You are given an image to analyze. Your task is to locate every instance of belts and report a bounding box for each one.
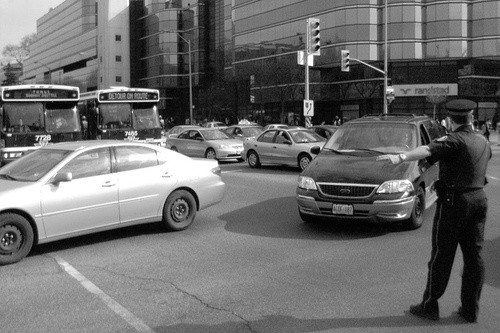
[455,188,478,193]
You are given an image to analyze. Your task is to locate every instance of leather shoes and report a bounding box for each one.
[409,304,439,321]
[458,306,477,323]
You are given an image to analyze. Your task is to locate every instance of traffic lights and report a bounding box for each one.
[308,17,320,56]
[340,49,350,72]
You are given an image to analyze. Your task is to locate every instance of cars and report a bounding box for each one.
[162,123,343,173]
[0,138,226,264]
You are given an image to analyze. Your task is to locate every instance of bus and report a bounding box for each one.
[77,84,164,148]
[0,83,89,167]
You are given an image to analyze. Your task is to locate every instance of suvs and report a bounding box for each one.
[294,111,453,232]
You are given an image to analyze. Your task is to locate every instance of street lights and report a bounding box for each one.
[80,52,99,90]
[33,61,51,84]
[166,31,193,126]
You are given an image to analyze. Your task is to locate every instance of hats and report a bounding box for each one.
[440,98,478,117]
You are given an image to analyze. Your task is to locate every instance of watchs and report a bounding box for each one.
[399,153,406,161]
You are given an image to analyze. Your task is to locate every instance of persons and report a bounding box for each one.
[255,112,304,127]
[422,113,492,145]
[185,115,230,126]
[158,112,175,132]
[376,100,492,322]
[334,115,341,125]
